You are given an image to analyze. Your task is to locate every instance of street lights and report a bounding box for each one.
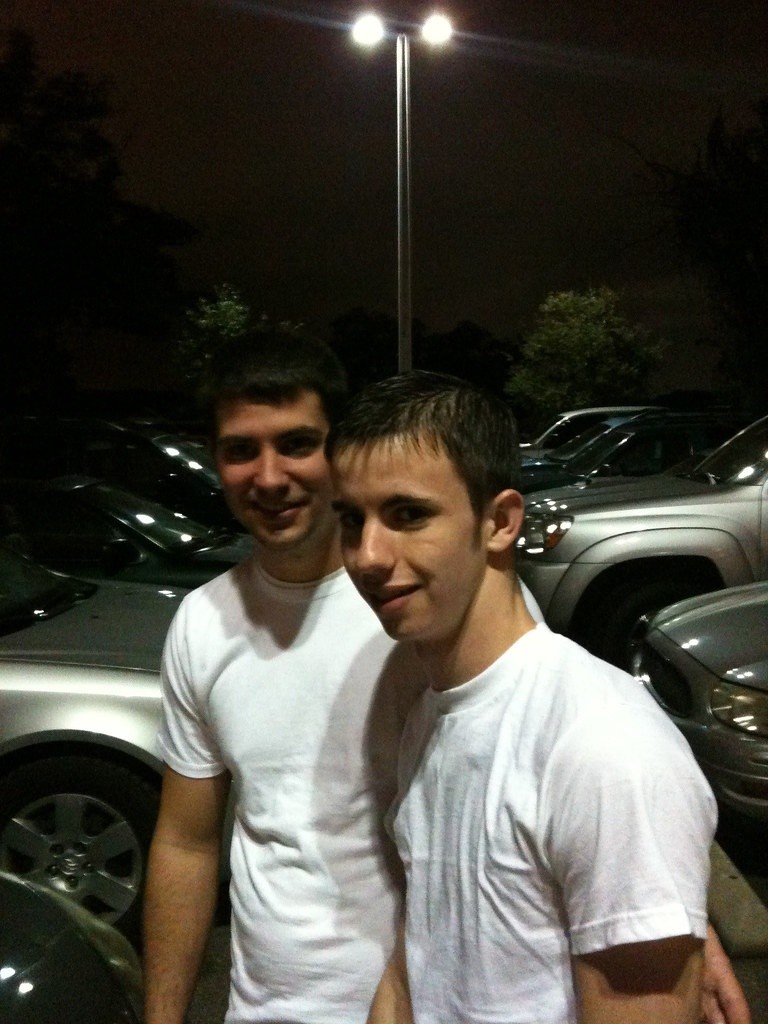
[352,3,460,373]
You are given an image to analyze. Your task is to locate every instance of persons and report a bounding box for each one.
[142,347,753,1024]
[325,370,720,1024]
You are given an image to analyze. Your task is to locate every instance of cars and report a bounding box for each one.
[630,577,768,836]
[514,403,768,675]
[1,402,267,1024]
